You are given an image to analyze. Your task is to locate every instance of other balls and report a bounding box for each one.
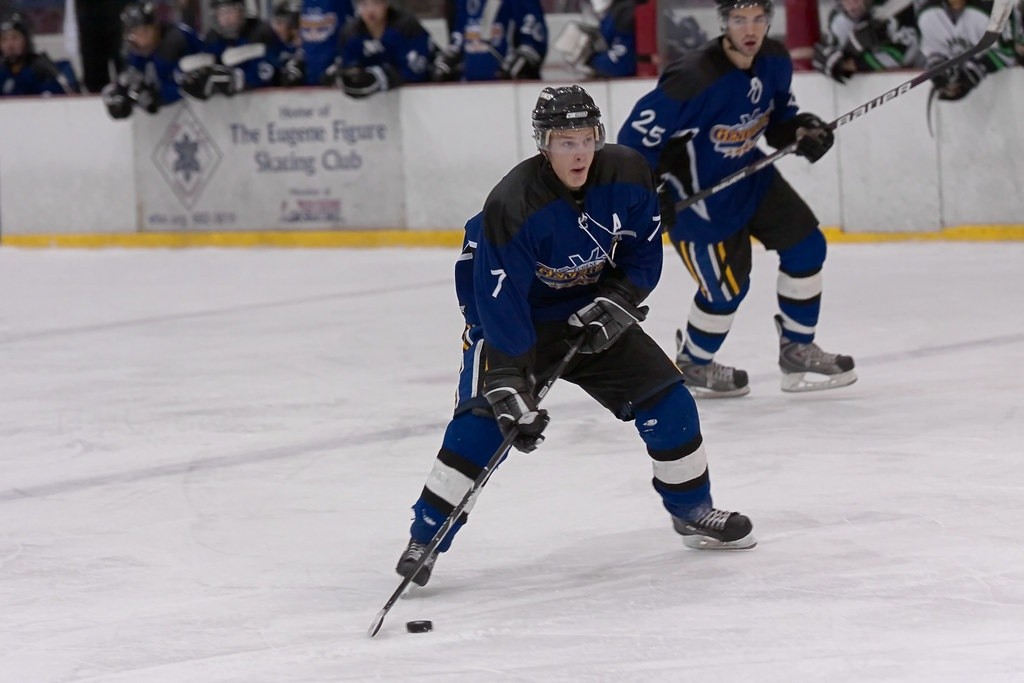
[406,620,433,633]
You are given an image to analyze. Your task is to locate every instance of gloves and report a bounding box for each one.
[432,56,462,81]
[566,293,649,355]
[184,65,232,100]
[928,53,983,102]
[502,47,542,79]
[481,368,549,453]
[104,72,159,120]
[767,104,835,163]
[652,172,675,227]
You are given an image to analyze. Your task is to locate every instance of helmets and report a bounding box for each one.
[715,0,774,35]
[208,0,244,37]
[120,0,160,58]
[532,84,608,161]
[0,9,33,65]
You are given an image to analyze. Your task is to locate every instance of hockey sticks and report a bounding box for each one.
[676,0,1014,213]
[368,330,588,638]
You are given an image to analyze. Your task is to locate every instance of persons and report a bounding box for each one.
[102,0,1024,122]
[396,85,754,586]
[0,18,81,99]
[617,0,856,392]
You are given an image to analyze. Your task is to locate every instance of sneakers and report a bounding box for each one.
[395,535,439,598]
[674,328,752,398]
[340,68,376,96]
[774,312,858,392]
[672,494,756,552]
